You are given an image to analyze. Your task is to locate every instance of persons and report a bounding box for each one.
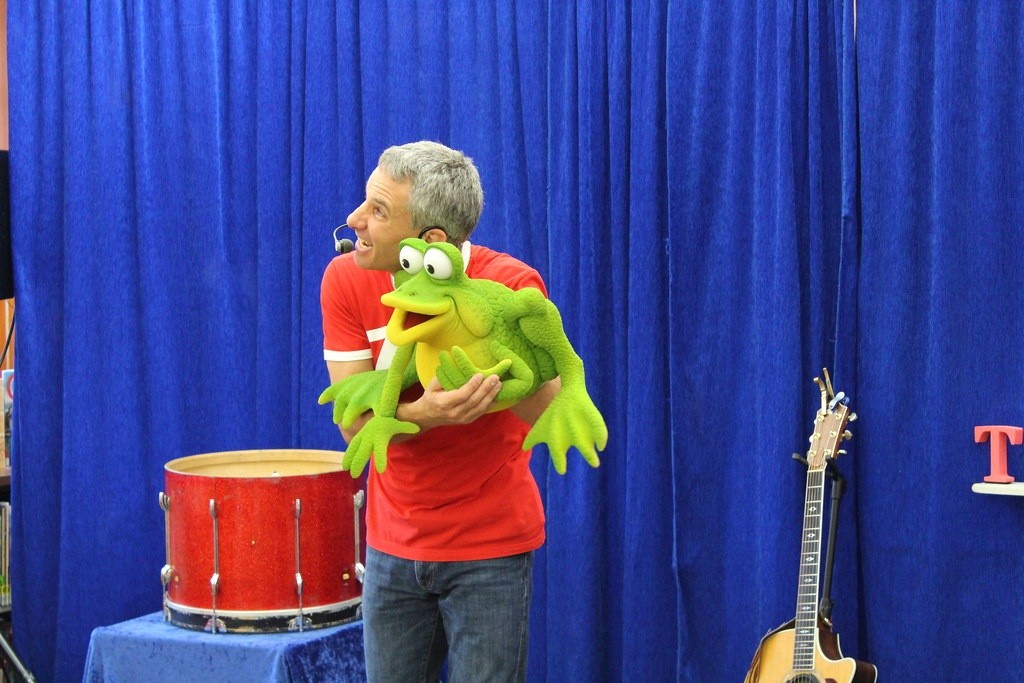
[320,140,562,683]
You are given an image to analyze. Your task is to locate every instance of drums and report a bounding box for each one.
[159,449,367,635]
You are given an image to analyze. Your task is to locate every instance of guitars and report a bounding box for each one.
[744,367,879,683]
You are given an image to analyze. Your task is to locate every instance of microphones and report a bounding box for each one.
[332,223,354,253]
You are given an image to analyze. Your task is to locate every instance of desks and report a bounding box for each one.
[82,607,368,682]
